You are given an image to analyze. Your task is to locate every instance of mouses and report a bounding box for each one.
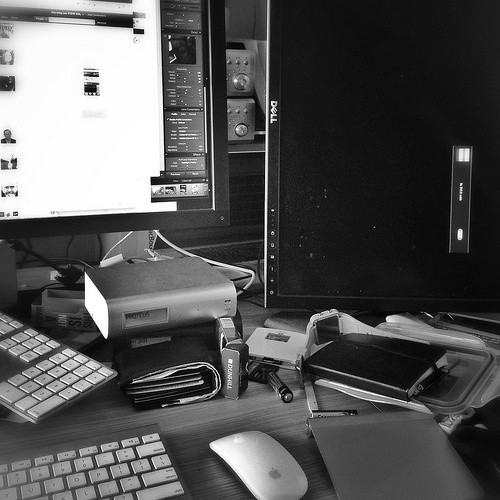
[207,431,309,500]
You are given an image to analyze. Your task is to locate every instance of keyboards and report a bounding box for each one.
[0,311,118,423]
[0,423,193,500]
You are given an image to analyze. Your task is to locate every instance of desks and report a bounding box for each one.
[0,297,499,500]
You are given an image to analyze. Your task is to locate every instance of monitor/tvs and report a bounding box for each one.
[264,0,500,312]
[0,0,231,264]
